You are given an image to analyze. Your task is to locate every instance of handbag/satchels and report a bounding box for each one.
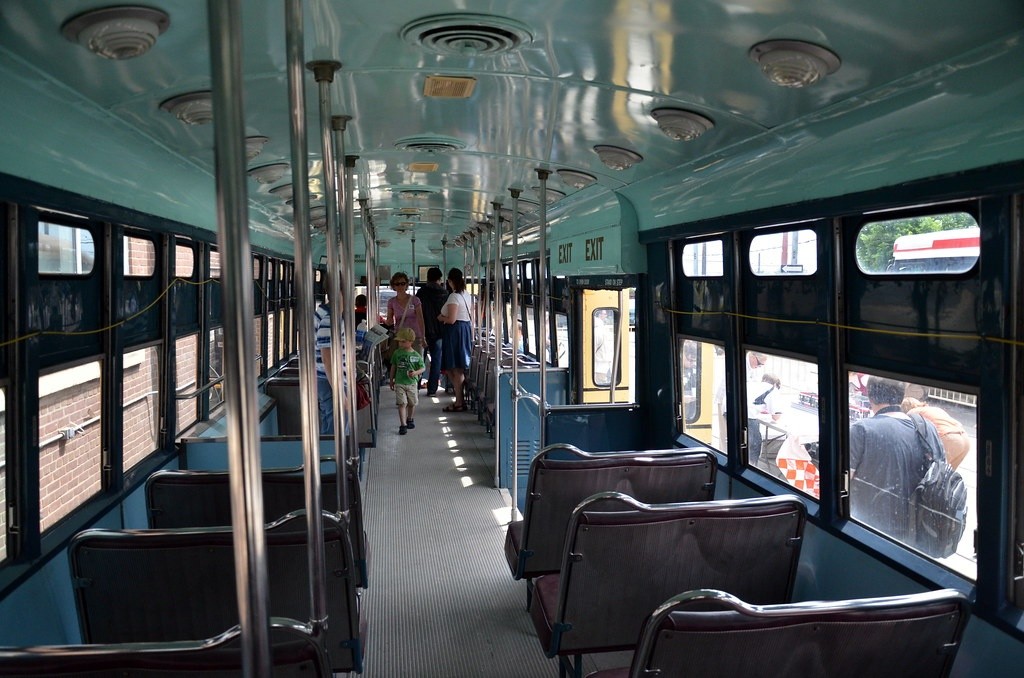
[343,382,370,412]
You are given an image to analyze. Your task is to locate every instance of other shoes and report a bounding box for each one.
[406,417,415,429]
[462,404,467,410]
[399,424,408,435]
[443,403,463,412]
[427,391,436,397]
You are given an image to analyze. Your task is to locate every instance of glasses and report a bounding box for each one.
[394,282,407,286]
[755,357,763,367]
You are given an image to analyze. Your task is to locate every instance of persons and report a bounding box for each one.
[849,375,946,543]
[716,350,768,453]
[437,267,471,412]
[747,372,781,466]
[901,397,971,471]
[415,268,449,396]
[382,272,428,404]
[389,327,426,434]
[313,276,350,435]
[354,295,383,323]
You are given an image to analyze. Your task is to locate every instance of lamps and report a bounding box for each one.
[532,187,566,204]
[247,163,290,184]
[158,90,213,126]
[59,4,171,60]
[650,107,715,143]
[748,39,841,86]
[556,169,597,188]
[246,136,269,161]
[593,145,643,171]
[268,184,292,196]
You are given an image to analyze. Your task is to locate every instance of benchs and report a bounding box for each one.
[0,356,380,678]
[468,338,970,678]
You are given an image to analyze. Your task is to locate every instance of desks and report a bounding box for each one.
[758,407,818,476]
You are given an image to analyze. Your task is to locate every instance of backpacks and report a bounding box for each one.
[907,412,969,560]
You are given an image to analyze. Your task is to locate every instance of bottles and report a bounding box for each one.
[356,319,367,346]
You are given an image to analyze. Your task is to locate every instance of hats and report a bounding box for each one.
[393,328,415,341]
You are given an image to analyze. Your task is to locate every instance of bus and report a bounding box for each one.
[490,287,714,446]
